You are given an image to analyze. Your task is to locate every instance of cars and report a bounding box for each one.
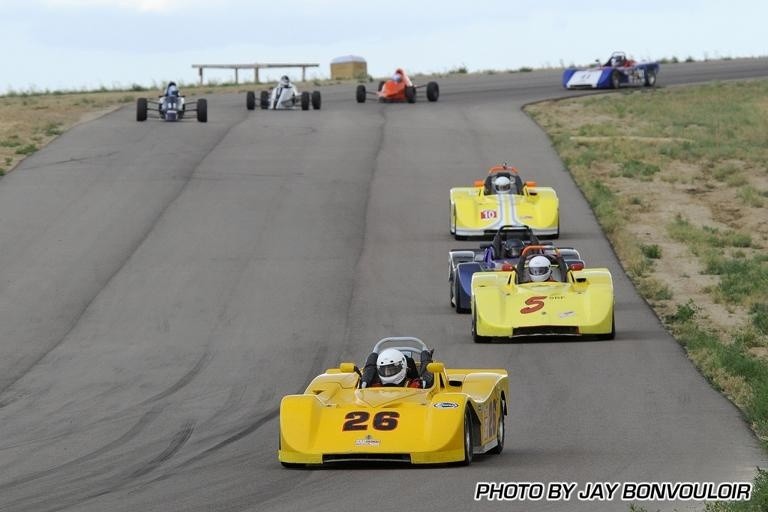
[277,336,510,470]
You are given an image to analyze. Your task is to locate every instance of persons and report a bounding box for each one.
[493,176,513,194]
[277,76,295,88]
[519,256,558,283]
[394,68,412,85]
[164,82,179,97]
[368,347,420,390]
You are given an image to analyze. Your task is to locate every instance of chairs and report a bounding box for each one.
[488,172,554,282]
[373,356,420,382]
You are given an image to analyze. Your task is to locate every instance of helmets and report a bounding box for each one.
[495,176,512,194]
[376,349,409,386]
[528,255,552,282]
[279,74,289,86]
[168,85,178,97]
